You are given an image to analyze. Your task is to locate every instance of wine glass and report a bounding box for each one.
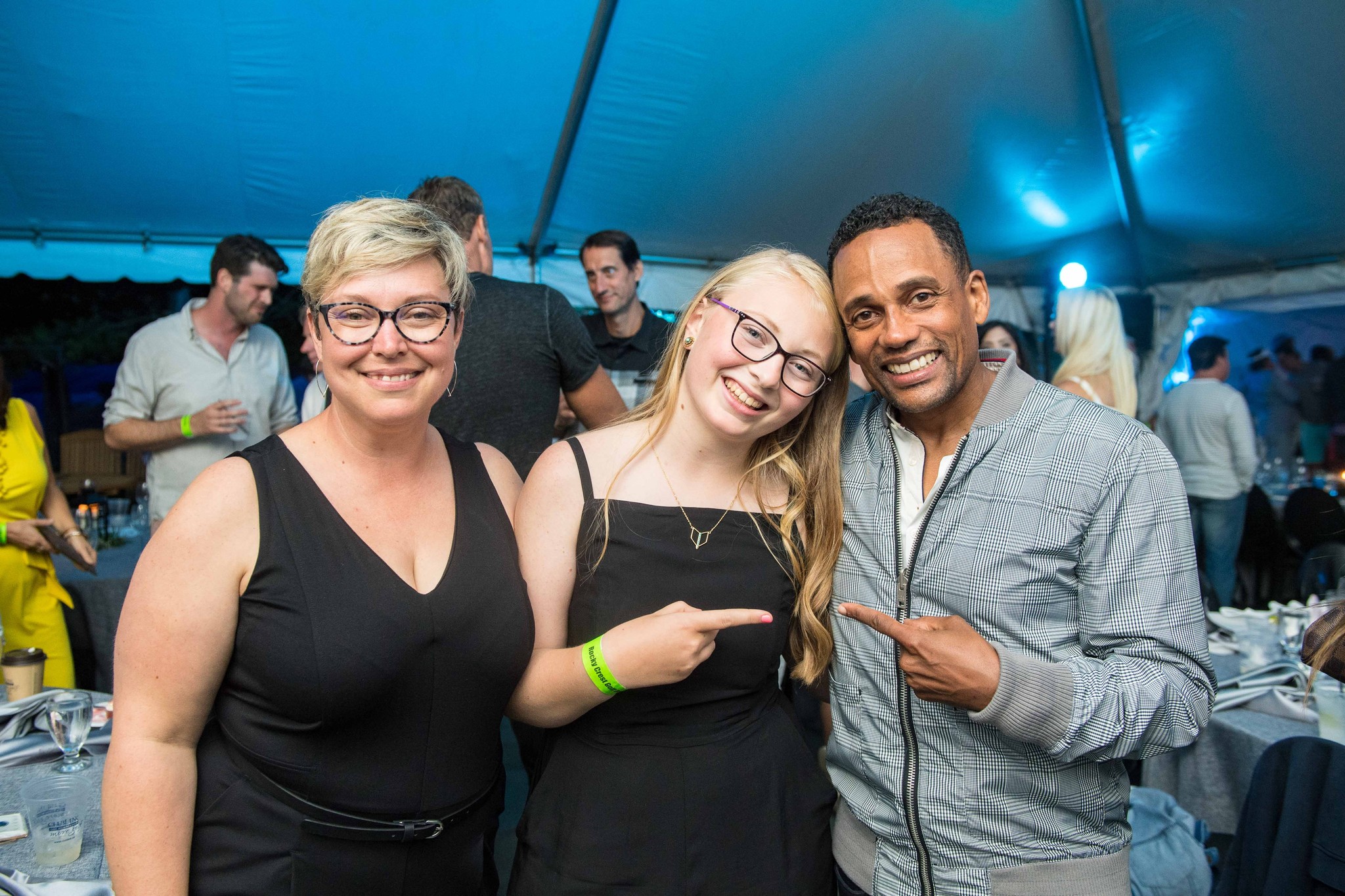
[1278,607,1310,659]
[45,691,94,774]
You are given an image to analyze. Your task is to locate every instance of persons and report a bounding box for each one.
[978,318,1029,372]
[101,228,301,545]
[1199,482,1345,613]
[552,229,678,437]
[1155,337,1261,608]
[290,305,333,424]
[406,177,632,480]
[0,375,96,692]
[1045,282,1140,416]
[1246,332,1344,479]
[826,195,1215,895]
[516,246,850,896]
[97,197,524,896]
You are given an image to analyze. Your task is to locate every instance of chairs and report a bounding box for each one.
[1238,481,1345,610]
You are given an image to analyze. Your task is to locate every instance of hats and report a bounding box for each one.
[1246,345,1272,363]
[1268,333,1295,354]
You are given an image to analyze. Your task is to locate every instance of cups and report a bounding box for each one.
[19,774,93,867]
[107,499,130,528]
[0,646,48,704]
[1311,679,1345,746]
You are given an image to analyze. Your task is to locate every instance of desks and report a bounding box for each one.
[39,509,150,695]
[1141,653,1345,834]
[0,684,115,896]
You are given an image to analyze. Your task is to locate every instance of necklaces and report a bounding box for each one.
[646,413,756,549]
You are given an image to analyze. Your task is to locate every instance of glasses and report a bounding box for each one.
[705,297,832,399]
[313,300,461,346]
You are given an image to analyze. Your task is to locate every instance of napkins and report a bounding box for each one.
[1206,594,1327,723]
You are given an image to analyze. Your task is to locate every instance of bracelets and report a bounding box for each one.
[581,632,629,698]
[177,414,197,440]
[59,525,86,540]
[0,523,8,545]
